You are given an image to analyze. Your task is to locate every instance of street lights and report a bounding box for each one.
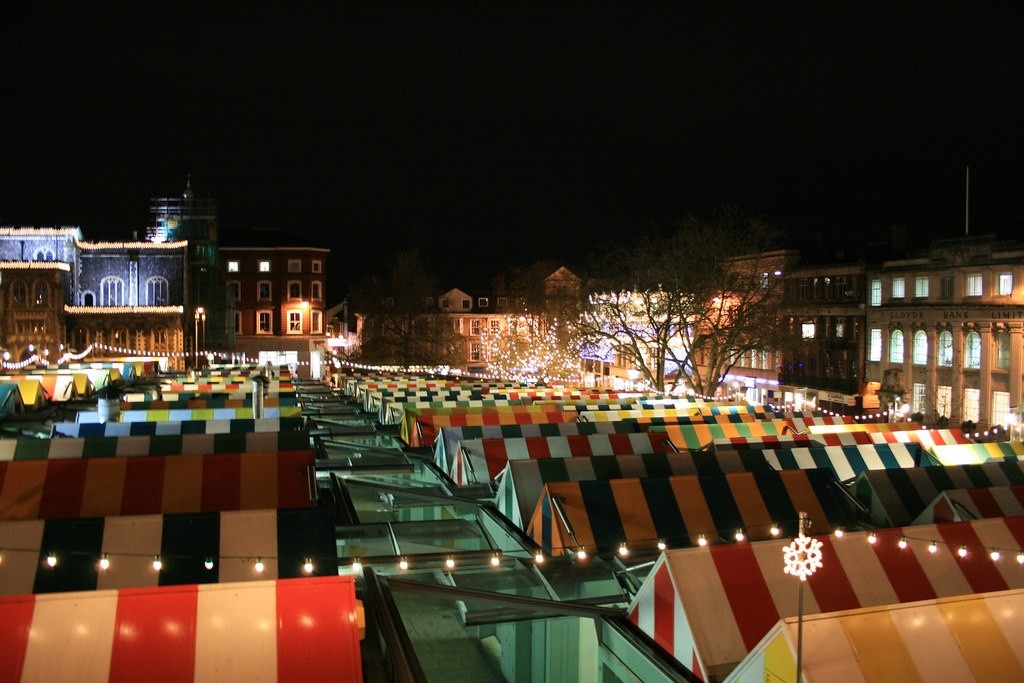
[195,307,206,372]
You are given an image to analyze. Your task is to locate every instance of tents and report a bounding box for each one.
[0,360,1024,683]
